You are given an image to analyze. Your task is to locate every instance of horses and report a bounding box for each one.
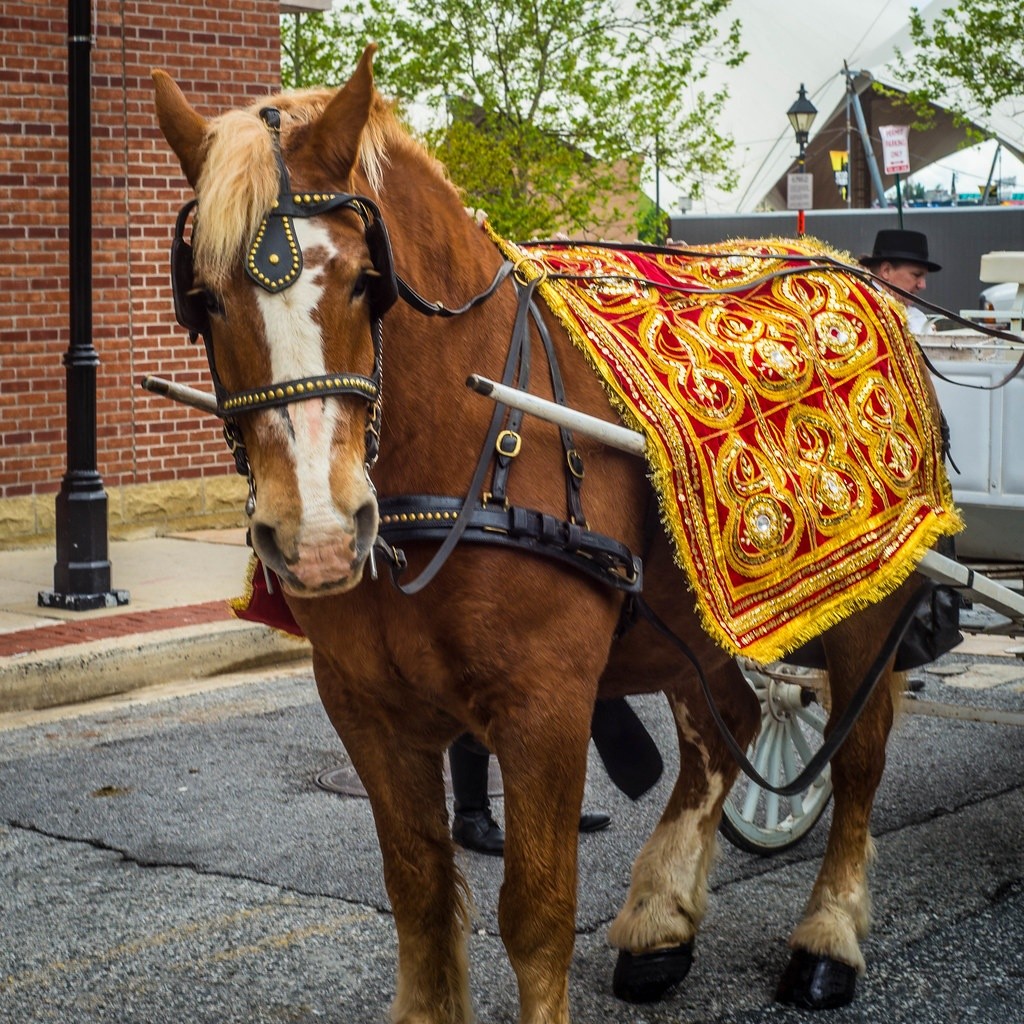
[151,43,942,1024]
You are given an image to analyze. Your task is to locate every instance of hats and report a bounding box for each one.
[858,229,943,273]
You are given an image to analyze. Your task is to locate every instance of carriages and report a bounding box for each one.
[144,39,1024,1024]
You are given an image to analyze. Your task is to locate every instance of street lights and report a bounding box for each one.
[786,81,819,173]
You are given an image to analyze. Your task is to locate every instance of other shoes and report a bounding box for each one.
[578,811,612,832]
[452,797,506,857]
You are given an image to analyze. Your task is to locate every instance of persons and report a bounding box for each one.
[447,732,612,858]
[855,230,942,336]
